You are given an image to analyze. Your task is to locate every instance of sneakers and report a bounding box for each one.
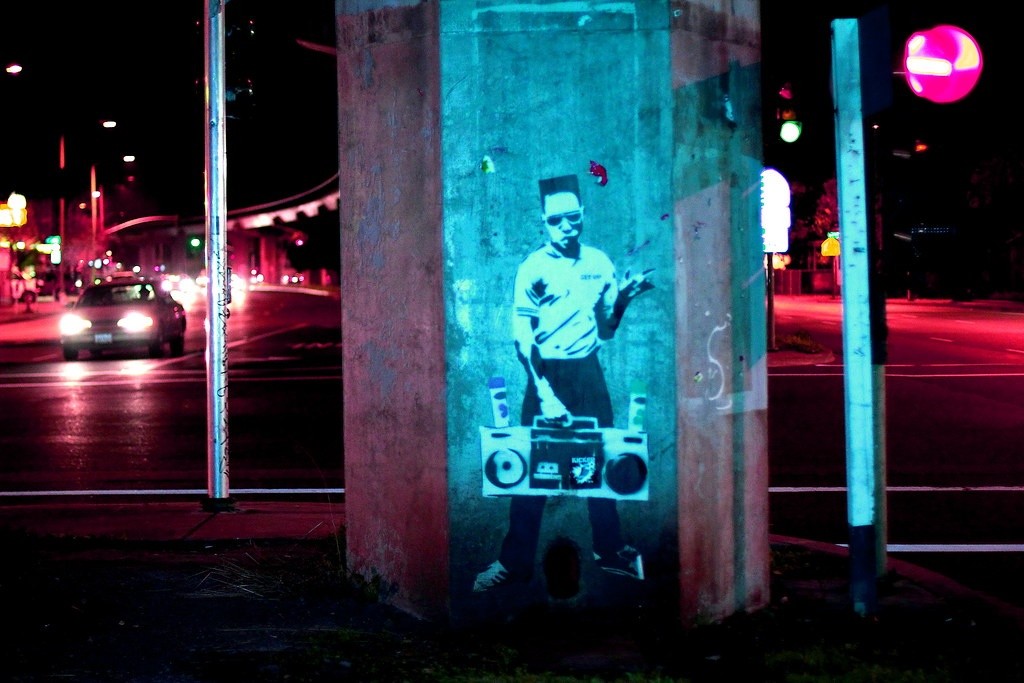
[593,545,644,580]
[475,560,510,590]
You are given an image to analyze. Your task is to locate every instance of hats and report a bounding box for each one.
[538,174,582,213]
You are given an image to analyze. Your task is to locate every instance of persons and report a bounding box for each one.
[471,174,656,595]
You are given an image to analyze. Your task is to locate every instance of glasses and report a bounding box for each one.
[544,208,582,226]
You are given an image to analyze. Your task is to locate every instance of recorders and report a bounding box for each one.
[478,425,649,500]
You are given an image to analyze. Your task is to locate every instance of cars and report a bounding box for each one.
[60,274,188,359]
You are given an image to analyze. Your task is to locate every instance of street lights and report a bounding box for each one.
[59,114,118,237]
